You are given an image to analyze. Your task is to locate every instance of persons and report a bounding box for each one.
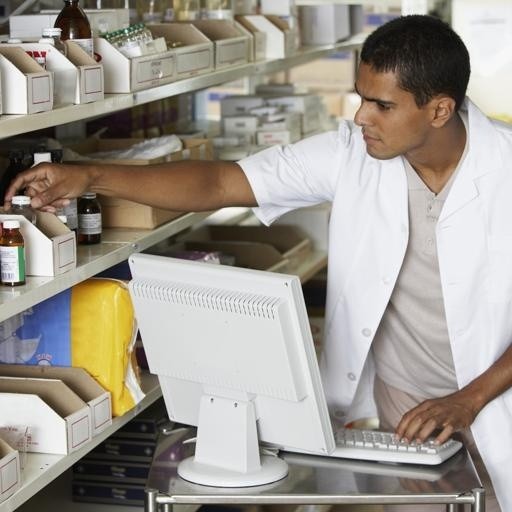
[4,14,512,512]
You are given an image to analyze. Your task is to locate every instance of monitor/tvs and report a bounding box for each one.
[127,251,336,488]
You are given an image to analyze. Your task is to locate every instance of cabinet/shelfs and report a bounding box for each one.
[1,0,362,512]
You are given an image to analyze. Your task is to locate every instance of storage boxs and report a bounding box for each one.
[60,134,213,228]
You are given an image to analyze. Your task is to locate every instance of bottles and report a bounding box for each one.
[163,0,176,23]
[79,194,102,246]
[140,0,163,24]
[8,196,36,227]
[49,149,78,237]
[173,1,199,21]
[207,0,233,26]
[3,148,30,195]
[54,0,93,58]
[103,22,153,52]
[0,220,26,287]
[39,28,67,57]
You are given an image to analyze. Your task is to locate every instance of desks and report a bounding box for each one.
[147,426,485,512]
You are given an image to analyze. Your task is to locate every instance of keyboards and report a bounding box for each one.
[331,426,463,466]
